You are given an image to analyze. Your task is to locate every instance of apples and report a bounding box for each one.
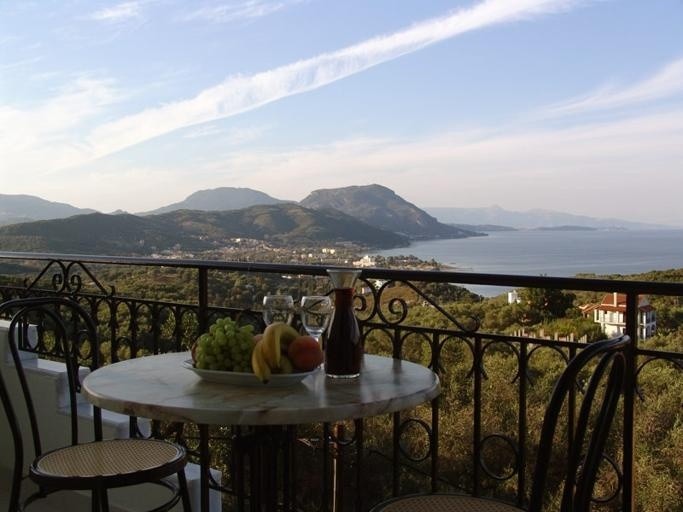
[289,336,321,371]
[191,336,199,362]
[254,335,263,344]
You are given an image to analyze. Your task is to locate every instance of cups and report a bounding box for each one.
[262,295,294,332]
[325,269,364,379]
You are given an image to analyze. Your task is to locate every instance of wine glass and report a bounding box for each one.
[300,296,331,371]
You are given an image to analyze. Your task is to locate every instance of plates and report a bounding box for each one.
[182,356,318,390]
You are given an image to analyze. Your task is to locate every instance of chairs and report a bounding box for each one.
[0,294,185,512]
[366,332,633,508]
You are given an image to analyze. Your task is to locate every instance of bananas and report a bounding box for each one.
[252,322,300,385]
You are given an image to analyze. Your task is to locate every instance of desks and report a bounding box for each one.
[81,345,442,512]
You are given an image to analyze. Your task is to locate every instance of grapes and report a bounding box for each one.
[194,317,255,373]
